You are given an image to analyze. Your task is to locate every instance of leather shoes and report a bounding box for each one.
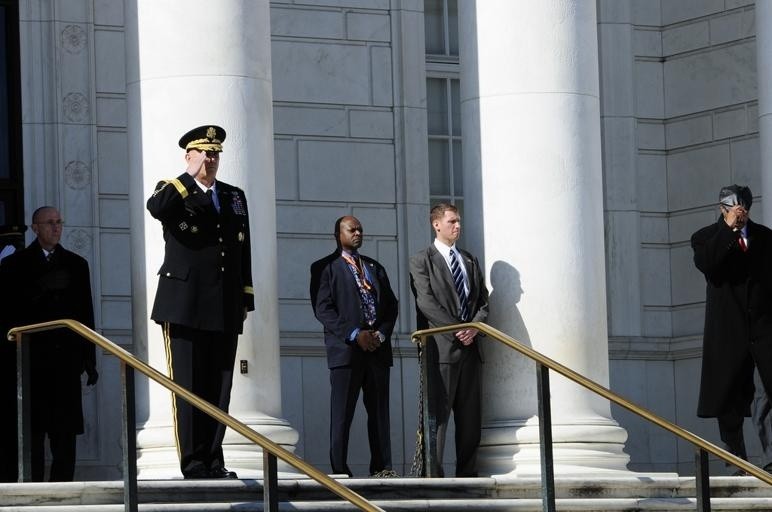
[86,366,99,387]
[0,244,16,261]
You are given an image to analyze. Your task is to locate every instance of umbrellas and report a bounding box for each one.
[209,467,237,480]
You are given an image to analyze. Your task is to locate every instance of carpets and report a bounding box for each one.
[205,189,216,210]
[351,259,377,324]
[448,249,469,320]
[45,252,55,263]
[735,230,748,252]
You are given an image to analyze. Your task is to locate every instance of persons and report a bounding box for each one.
[1,220,27,262]
[685,182,772,478]
[306,210,402,479]
[0,203,100,485]
[406,199,492,478]
[145,119,259,480]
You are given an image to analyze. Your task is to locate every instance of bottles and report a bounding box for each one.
[178,124,227,154]
[1,224,29,240]
[719,183,753,215]
